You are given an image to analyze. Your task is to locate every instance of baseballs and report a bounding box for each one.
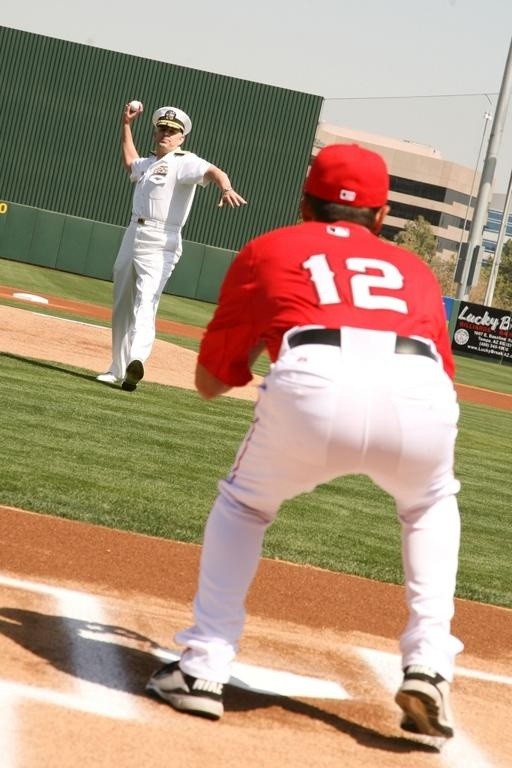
[129,100,140,112]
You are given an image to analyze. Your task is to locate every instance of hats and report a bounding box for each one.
[152,106,192,135]
[302,144,390,208]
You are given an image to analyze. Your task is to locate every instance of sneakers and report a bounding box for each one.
[145,659,224,721]
[96,360,144,392]
[395,663,454,739]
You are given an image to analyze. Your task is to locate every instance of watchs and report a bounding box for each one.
[220,184,232,194]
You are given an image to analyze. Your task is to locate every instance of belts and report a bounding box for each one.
[287,329,436,362]
[131,215,181,233]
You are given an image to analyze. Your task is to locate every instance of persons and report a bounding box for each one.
[93,102,250,392]
[145,142,466,739]
